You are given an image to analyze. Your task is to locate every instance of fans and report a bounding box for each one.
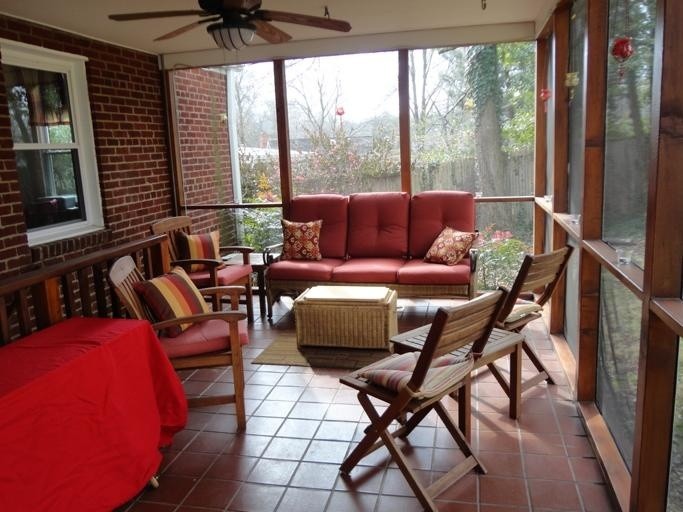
[107,0,352,46]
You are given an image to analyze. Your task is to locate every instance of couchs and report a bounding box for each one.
[263,192,478,322]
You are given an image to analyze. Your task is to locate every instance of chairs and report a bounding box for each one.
[337,284,508,511]
[449,244,576,421]
[150,215,256,321]
[106,254,247,435]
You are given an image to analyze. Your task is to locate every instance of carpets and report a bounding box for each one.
[251,330,391,369]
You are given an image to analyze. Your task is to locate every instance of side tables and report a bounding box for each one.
[391,319,525,452]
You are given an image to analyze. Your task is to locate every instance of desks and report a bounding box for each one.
[0,316,187,511]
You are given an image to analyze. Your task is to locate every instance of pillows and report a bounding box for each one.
[131,265,212,339]
[422,226,478,265]
[280,219,322,261]
[177,227,220,273]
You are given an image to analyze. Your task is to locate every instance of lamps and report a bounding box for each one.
[207,11,256,50]
